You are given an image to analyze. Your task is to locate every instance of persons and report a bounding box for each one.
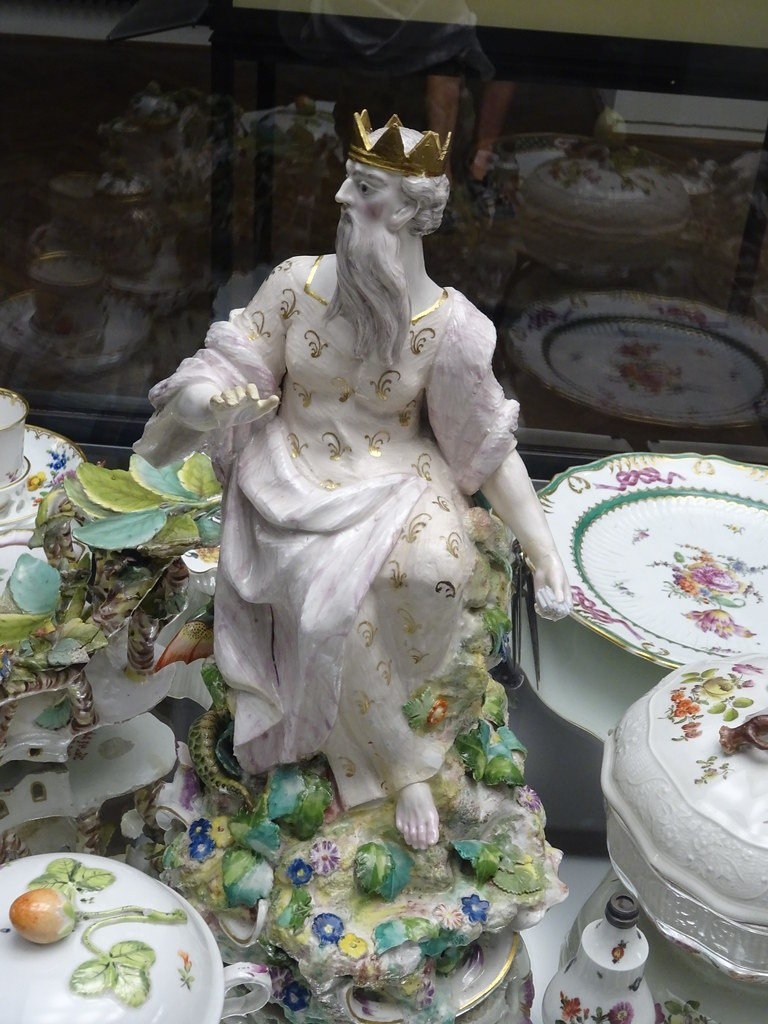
[151,116,576,851]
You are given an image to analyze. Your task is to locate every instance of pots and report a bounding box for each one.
[511,147,692,278]
[600,652,768,988]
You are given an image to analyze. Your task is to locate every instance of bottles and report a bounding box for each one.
[541,894,656,1024]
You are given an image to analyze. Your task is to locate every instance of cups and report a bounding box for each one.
[0,387,30,488]
[0,852,273,1024]
[27,252,110,335]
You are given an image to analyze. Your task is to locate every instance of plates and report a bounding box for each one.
[28,309,108,356]
[0,289,152,375]
[0,425,87,526]
[504,291,768,428]
[517,452,768,671]
[0,454,32,513]
